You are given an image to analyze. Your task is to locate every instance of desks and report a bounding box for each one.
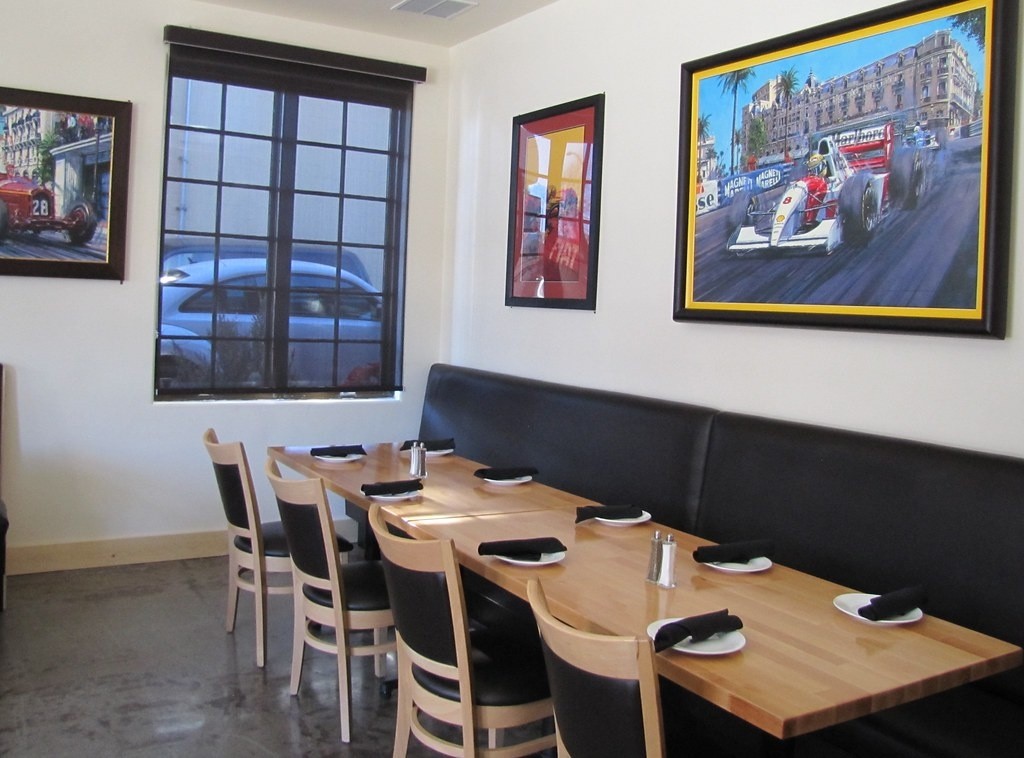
[267,446,605,530]
[405,504,1024,741]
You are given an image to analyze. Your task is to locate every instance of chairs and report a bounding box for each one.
[364,504,557,757]
[528,579,667,758]
[202,429,351,668]
[264,458,396,743]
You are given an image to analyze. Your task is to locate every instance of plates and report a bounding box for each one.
[482,475,532,487]
[833,593,923,627]
[703,556,773,575]
[647,617,746,655]
[493,552,566,567]
[593,509,651,528]
[370,490,421,502]
[314,454,363,464]
[426,449,454,458]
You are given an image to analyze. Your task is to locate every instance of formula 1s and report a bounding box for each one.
[723,120,929,258]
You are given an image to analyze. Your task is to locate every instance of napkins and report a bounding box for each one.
[311,444,368,457]
[362,479,423,496]
[475,466,539,481]
[476,536,566,562]
[400,437,454,452]
[692,538,776,563]
[575,504,642,522]
[858,582,931,623]
[653,608,744,654]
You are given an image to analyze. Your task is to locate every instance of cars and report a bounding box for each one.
[1,166,99,248]
[161,257,382,384]
[160,325,224,391]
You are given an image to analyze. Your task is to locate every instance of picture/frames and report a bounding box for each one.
[504,92,608,309]
[0,84,133,281]
[674,0,1018,340]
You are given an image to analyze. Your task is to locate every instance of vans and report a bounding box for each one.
[161,238,375,284]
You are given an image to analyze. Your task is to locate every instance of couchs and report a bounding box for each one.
[418,364,1024,756]
